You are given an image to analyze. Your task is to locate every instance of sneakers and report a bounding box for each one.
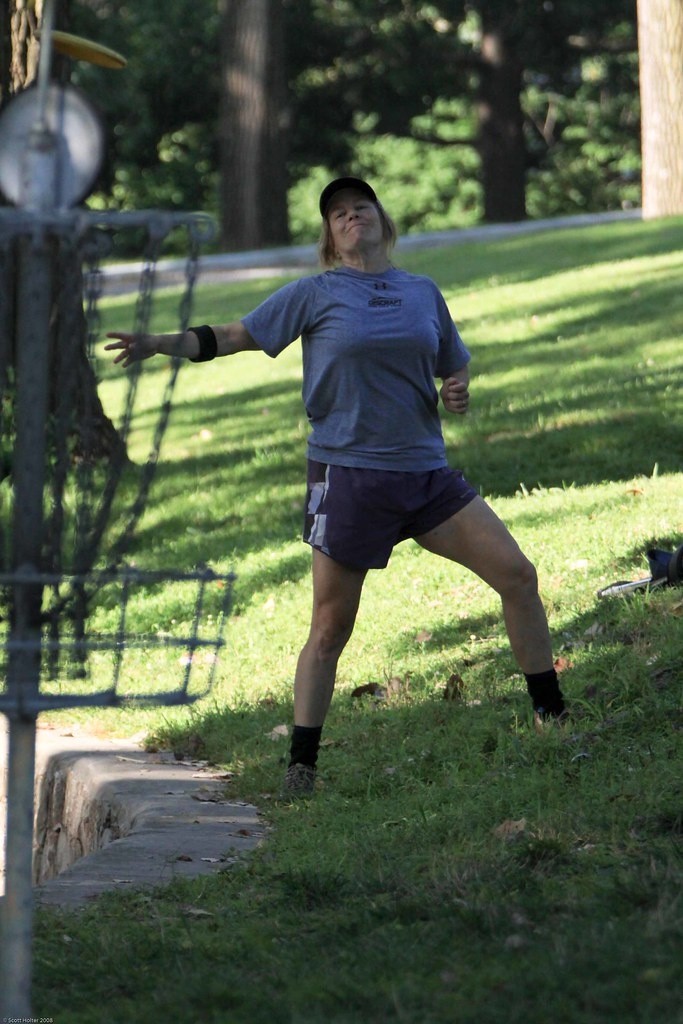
[280,765,317,805]
[537,705,586,732]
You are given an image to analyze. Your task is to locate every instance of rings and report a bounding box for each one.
[461,399,466,407]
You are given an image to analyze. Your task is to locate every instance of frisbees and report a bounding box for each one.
[29,28,125,69]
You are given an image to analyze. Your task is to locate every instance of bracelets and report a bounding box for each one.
[186,324,220,362]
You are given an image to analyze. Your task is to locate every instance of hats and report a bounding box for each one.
[320,178,375,217]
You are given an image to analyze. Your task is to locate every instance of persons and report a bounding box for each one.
[102,176,578,805]
